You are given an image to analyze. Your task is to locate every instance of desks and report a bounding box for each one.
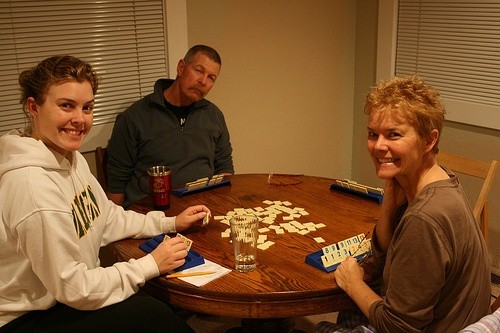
[109,173,393,333]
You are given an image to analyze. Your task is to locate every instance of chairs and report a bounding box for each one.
[436,151,500,248]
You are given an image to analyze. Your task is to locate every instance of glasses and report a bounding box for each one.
[351,231,372,266]
[268,173,304,186]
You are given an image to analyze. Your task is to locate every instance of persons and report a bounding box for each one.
[315,75,491,333]
[106,44,234,206]
[0,55,211,333]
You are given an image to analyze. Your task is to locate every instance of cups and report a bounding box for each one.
[229,215,259,273]
[146,166,172,211]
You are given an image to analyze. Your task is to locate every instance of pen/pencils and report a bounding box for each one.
[166,271,216,278]
